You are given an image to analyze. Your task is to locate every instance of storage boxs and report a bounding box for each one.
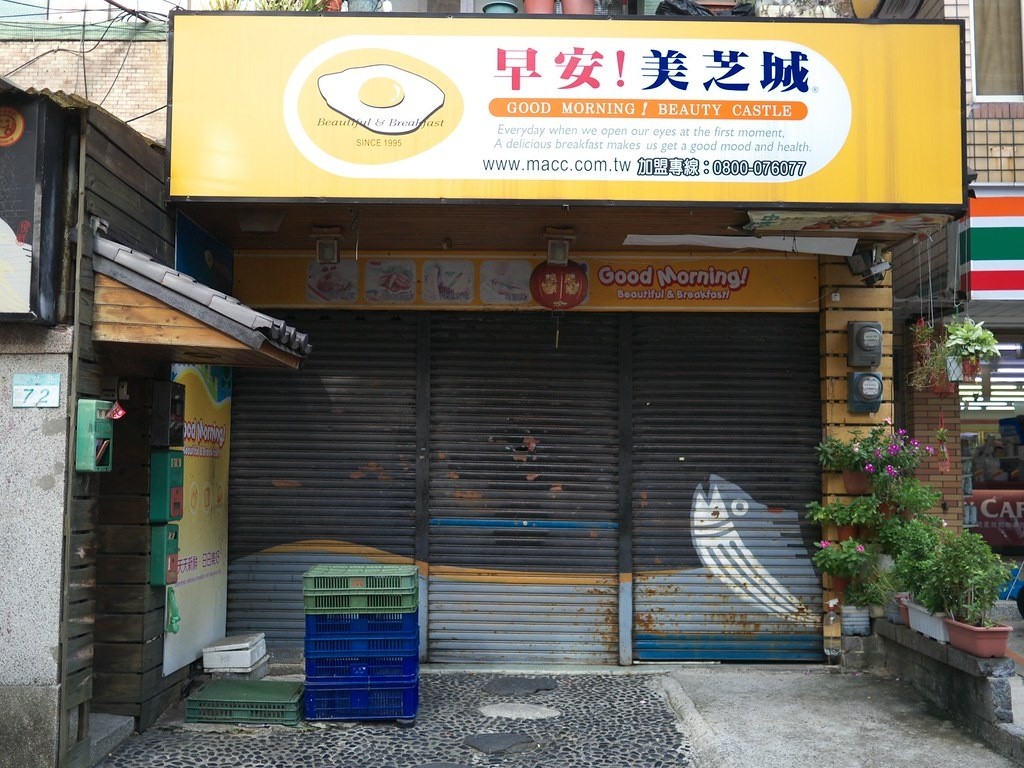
[185,563,420,727]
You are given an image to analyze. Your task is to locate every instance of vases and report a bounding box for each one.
[482,0,596,14]
[831,577,850,594]
[323,0,381,12]
[842,469,871,496]
[912,343,931,363]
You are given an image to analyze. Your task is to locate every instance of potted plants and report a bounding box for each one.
[905,312,1003,393]
[804,476,1024,658]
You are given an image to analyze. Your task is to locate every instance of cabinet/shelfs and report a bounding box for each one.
[961,457,980,527]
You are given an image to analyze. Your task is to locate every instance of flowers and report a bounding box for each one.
[910,318,935,343]
[812,535,872,578]
[863,427,936,497]
[816,416,893,473]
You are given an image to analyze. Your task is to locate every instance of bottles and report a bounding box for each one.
[823,608,841,656]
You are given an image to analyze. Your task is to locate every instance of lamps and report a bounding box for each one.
[543,227,577,267]
[309,226,347,264]
[847,242,894,287]
